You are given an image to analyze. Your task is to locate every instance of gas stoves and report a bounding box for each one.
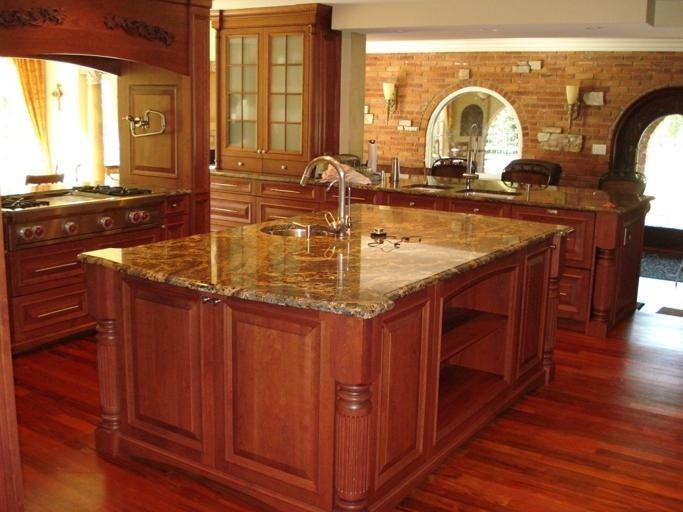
[0,184,169,253]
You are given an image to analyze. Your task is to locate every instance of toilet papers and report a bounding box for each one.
[367,142,377,174]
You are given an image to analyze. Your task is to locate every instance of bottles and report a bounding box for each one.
[360,138,401,187]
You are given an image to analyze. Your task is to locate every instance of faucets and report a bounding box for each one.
[300,155,347,235]
[462,123,479,190]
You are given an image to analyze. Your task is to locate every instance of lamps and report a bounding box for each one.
[565,86,579,133]
[382,83,397,125]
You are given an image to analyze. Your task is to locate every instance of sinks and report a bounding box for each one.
[399,183,456,192]
[455,189,522,198]
[259,224,345,237]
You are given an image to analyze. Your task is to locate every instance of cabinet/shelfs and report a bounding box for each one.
[209,3,341,178]
[7,196,198,357]
[212,179,596,330]
[370,241,560,499]
[122,277,330,509]
